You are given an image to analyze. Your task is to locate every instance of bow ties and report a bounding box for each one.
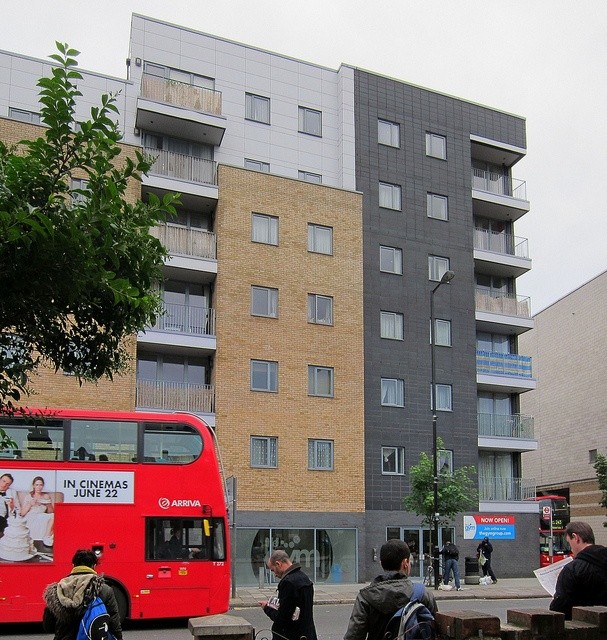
[0,492,6,497]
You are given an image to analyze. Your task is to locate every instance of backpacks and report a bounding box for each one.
[77,597,118,640]
[444,543,459,556]
[478,550,488,568]
[383,583,441,640]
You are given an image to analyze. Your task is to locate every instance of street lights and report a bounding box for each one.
[431,270,456,590]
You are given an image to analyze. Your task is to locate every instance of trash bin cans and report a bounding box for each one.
[464,556,480,585]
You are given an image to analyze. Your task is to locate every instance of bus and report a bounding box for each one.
[0,406,231,623]
[526,495,570,568]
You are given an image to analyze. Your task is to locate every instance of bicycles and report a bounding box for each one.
[422,552,453,587]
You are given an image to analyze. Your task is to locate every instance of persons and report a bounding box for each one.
[42,549,123,640]
[437,540,461,591]
[344,538,441,639]
[549,522,606,620]
[17,476,55,547]
[167,527,201,559]
[258,549,317,639]
[0,473,20,539]
[477,536,497,585]
